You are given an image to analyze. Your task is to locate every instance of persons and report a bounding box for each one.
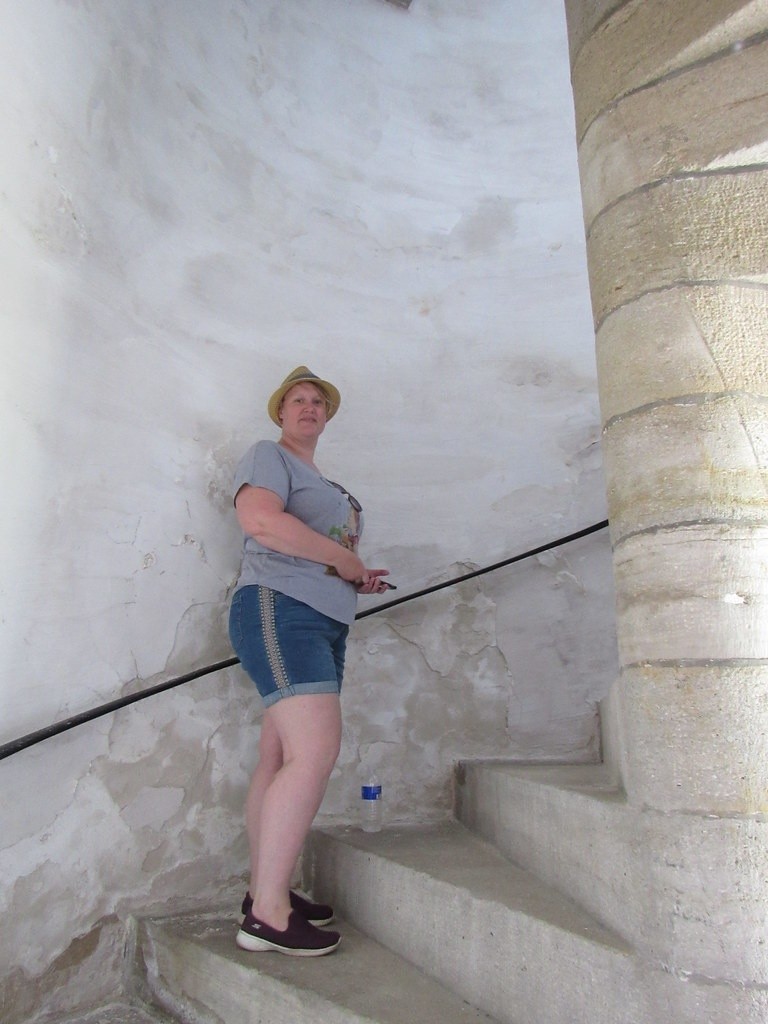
[228,365,390,957]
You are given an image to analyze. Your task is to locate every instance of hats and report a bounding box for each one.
[267,366,341,429]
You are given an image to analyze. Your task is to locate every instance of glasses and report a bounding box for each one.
[327,479,362,513]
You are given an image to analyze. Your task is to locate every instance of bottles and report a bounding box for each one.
[361,769,382,832]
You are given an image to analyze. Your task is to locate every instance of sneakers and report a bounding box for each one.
[236,907,342,958]
[238,888,333,927]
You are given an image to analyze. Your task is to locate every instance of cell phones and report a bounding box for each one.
[368,577,397,590]
